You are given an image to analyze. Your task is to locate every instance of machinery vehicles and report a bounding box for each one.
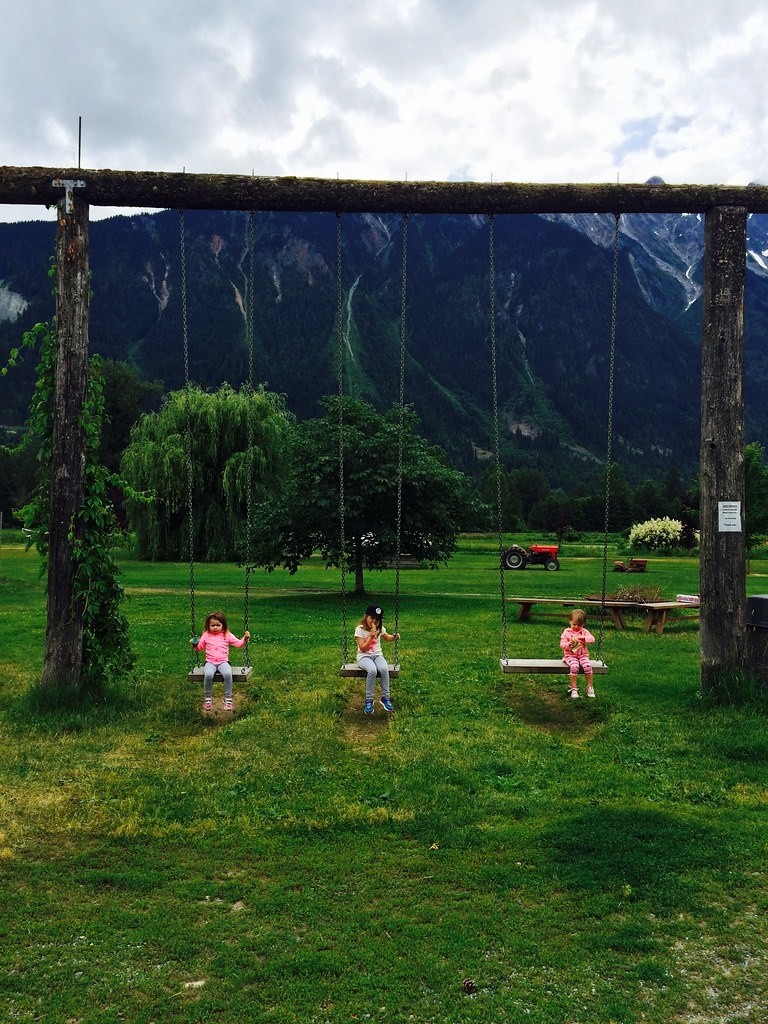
[500,544,560,571]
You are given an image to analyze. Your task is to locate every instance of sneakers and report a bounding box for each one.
[379,695,394,712]
[364,699,374,715]
[203,696,212,711]
[221,696,232,711]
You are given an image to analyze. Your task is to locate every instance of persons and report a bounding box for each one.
[189,611,250,712]
[354,606,401,715]
[560,609,596,698]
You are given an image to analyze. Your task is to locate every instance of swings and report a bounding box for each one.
[489,208,622,670]
[168,208,256,678]
[337,210,407,674]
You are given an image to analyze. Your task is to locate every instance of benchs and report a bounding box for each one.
[507,597,638,631]
[640,602,699,635]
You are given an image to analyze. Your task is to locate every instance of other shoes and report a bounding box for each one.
[585,685,596,698]
[567,687,580,699]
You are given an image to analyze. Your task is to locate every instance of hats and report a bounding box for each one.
[365,605,385,621]
